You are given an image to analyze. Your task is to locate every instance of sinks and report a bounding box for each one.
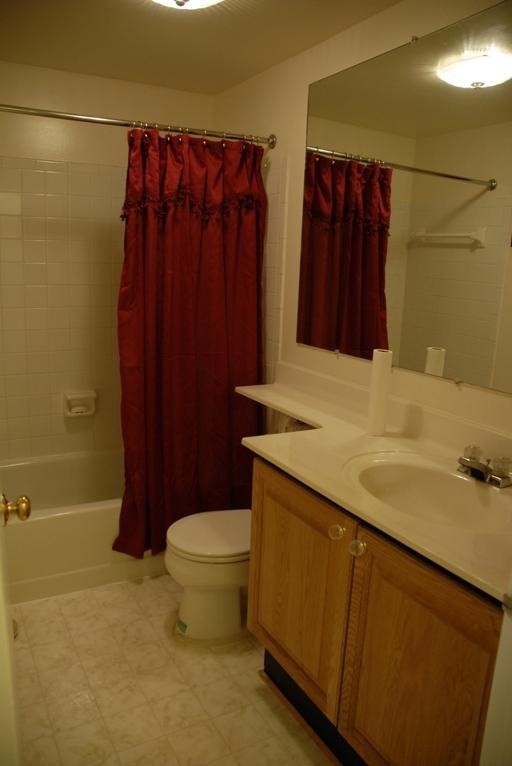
[342,451,512,532]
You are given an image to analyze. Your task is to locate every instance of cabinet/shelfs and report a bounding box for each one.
[246,460,512,766]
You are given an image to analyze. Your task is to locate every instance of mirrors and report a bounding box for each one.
[288,0,512,393]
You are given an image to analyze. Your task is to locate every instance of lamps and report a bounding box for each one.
[436,45,512,88]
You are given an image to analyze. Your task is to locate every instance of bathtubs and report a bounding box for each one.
[0,449,174,605]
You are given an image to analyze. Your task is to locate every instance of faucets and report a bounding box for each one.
[458,456,491,481]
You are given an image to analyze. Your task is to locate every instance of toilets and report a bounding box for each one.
[164,509,251,636]
